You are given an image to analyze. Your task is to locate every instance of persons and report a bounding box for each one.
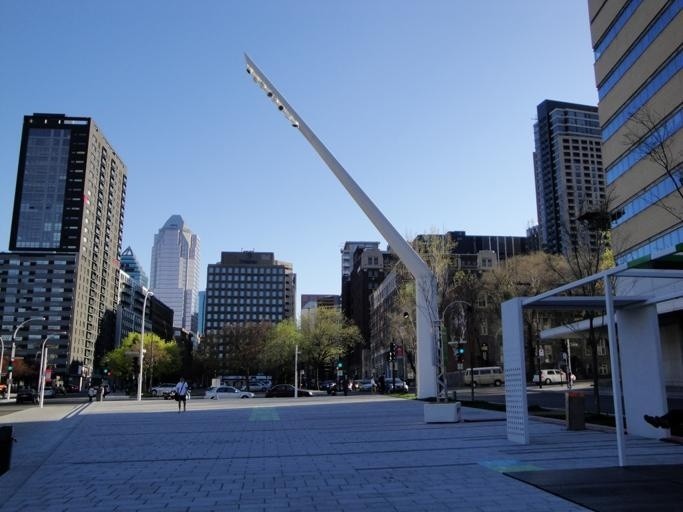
[643,403,682,429]
[87,384,94,402]
[99,384,104,402]
[301,372,388,396]
[174,376,190,413]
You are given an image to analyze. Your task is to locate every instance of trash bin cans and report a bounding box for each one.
[566,390,585,431]
[0,426,17,477]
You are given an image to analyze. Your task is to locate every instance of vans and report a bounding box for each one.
[465,366,504,388]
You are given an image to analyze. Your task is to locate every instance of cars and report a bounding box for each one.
[320,378,409,393]
[532,369,576,385]
[149,383,313,399]
[16,389,40,405]
[66,385,79,392]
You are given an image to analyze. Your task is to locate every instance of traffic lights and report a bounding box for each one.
[338,352,342,367]
[560,339,565,351]
[454,347,464,361]
[131,357,140,373]
[386,342,395,361]
[104,368,112,376]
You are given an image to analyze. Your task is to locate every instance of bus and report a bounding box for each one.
[217,376,272,392]
[91,376,109,395]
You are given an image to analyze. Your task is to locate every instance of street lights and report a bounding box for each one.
[383,324,405,394]
[6,317,66,402]
[441,301,471,373]
[137,292,154,401]
